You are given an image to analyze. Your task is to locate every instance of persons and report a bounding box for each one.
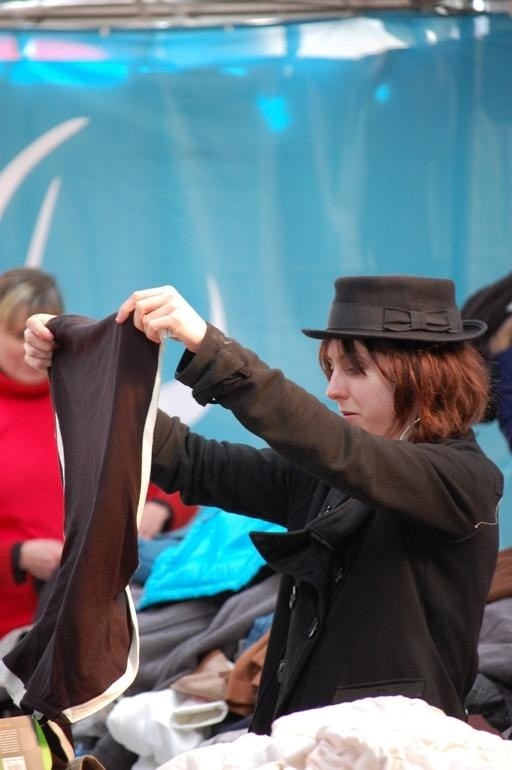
[459,274,512,457]
[0,268,199,726]
[21,273,505,742]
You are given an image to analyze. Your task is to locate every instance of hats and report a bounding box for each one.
[299,274,489,347]
